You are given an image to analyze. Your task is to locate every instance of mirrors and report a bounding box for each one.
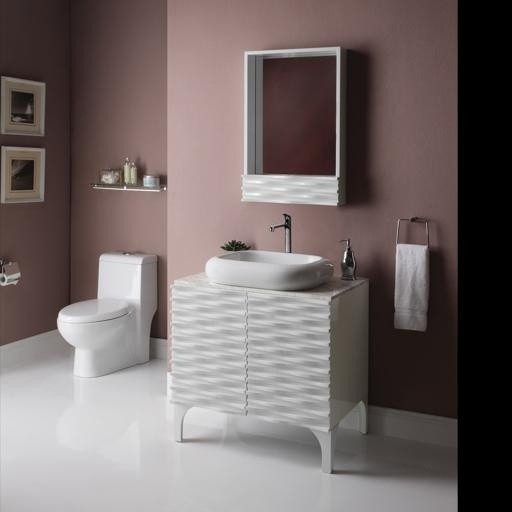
[241,46,347,208]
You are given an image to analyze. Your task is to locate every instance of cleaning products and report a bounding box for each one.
[124,157,137,185]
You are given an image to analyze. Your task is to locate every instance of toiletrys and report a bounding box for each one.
[129,162,138,185]
[123,157,131,183]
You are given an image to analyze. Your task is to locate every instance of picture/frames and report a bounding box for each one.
[0,76,46,204]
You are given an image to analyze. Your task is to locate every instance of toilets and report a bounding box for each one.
[57,251,158,377]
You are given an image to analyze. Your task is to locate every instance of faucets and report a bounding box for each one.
[270,213,291,253]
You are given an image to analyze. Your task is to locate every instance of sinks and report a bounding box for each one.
[205,250,333,290]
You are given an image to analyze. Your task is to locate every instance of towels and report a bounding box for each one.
[394,243,431,331]
